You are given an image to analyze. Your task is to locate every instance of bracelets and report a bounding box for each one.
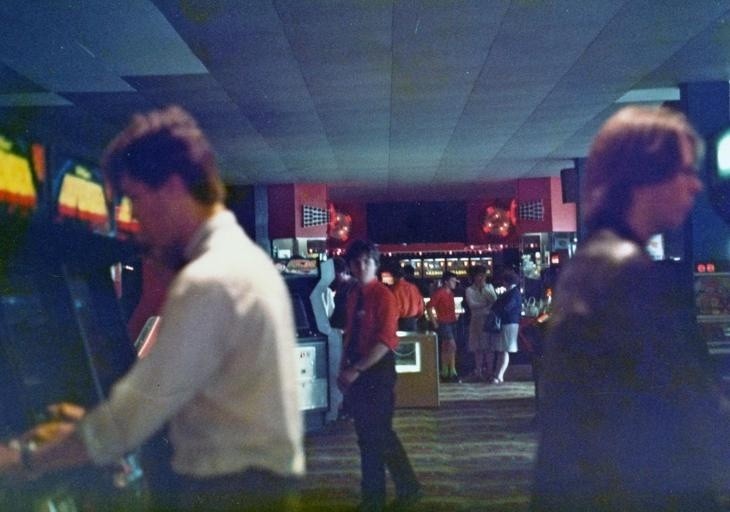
[350,365,364,374]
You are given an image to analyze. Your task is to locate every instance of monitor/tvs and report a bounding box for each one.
[469,256,493,276]
[446,257,470,278]
[423,257,446,278]
[398,258,422,278]
[291,292,309,330]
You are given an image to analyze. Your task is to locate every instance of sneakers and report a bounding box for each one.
[490,377,504,385]
[440,374,450,381]
[450,375,462,383]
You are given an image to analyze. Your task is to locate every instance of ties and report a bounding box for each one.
[348,288,363,365]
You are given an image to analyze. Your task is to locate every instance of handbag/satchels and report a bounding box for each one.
[482,310,501,333]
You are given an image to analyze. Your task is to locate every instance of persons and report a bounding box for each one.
[333,242,425,512]
[1,105,305,512]
[328,259,359,329]
[489,271,523,385]
[375,263,425,360]
[533,104,730,511]
[425,271,463,384]
[464,265,497,379]
